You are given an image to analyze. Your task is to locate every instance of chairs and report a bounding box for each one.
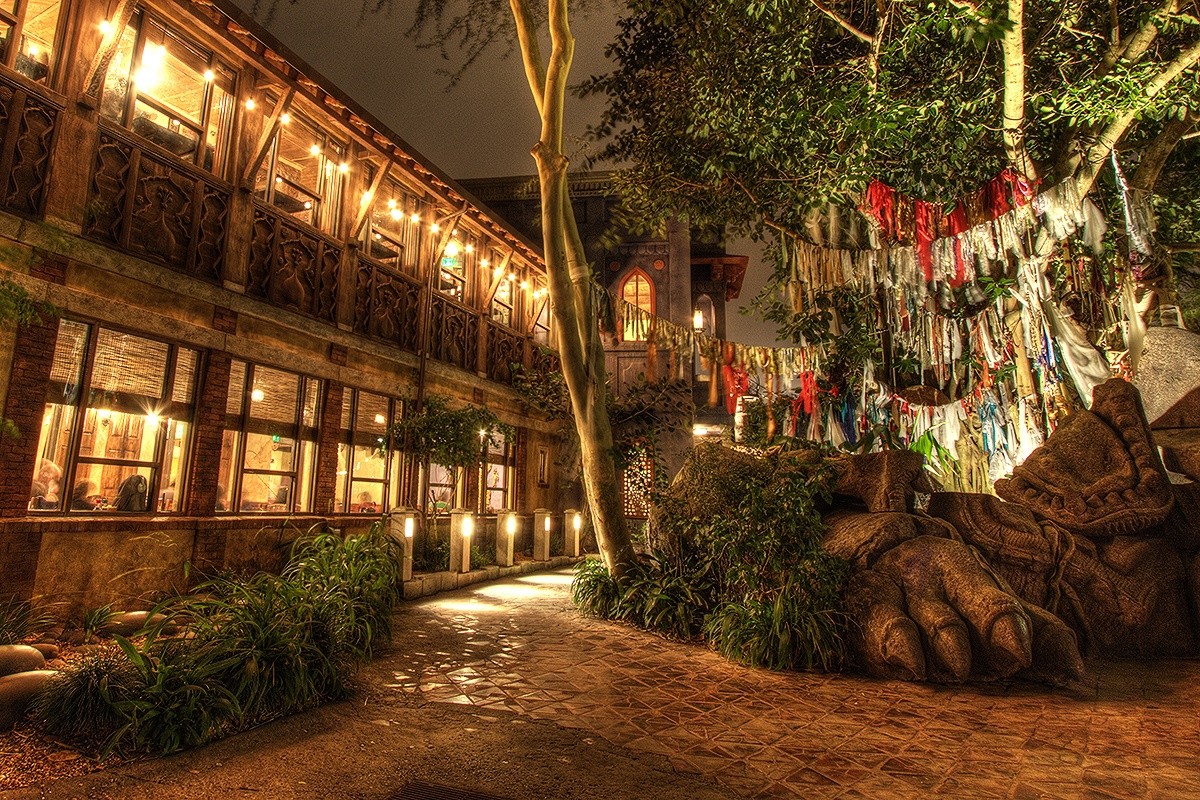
[192,142,214,172]
[387,262,396,269]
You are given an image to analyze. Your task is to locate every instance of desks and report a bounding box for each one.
[359,240,399,259]
[253,185,312,214]
[0,42,49,83]
[432,277,458,290]
[131,116,197,158]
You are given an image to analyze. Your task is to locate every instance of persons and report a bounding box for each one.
[216,482,253,511]
[17,49,50,86]
[493,304,508,326]
[160,481,176,499]
[334,492,378,513]
[28,457,108,510]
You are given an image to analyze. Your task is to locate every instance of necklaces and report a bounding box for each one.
[272,486,289,509]
